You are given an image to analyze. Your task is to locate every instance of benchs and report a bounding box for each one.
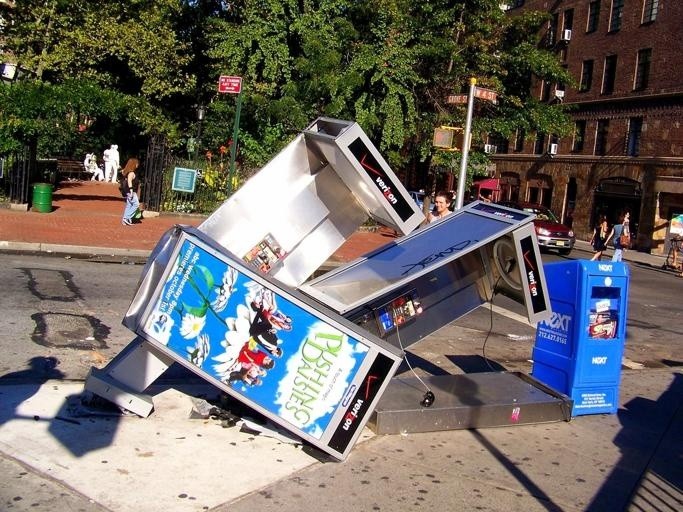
[56,160,94,180]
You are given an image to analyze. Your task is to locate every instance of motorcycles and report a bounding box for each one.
[662,236,683,276]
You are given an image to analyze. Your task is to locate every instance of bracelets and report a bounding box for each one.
[129,187,133,189]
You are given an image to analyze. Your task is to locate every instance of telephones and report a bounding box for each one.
[423,390,435,407]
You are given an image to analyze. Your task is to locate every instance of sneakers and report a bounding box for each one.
[122,218,135,226]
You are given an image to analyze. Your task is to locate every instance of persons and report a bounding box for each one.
[120,158,140,226]
[84,144,121,184]
[670,235,683,277]
[419,188,457,224]
[225,292,293,389]
[622,210,631,252]
[603,215,629,262]
[589,213,609,261]
[478,193,493,203]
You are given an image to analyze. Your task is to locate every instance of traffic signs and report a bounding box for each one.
[474,86,496,101]
[447,95,468,104]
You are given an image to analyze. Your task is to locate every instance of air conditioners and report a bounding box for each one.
[547,143,558,155]
[483,143,492,154]
[561,29,571,41]
[552,89,565,99]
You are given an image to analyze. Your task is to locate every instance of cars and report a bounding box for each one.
[495,199,575,255]
[408,190,436,213]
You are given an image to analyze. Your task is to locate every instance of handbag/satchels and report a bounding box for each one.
[619,225,631,246]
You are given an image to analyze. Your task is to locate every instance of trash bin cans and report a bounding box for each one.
[32,183,52,213]
